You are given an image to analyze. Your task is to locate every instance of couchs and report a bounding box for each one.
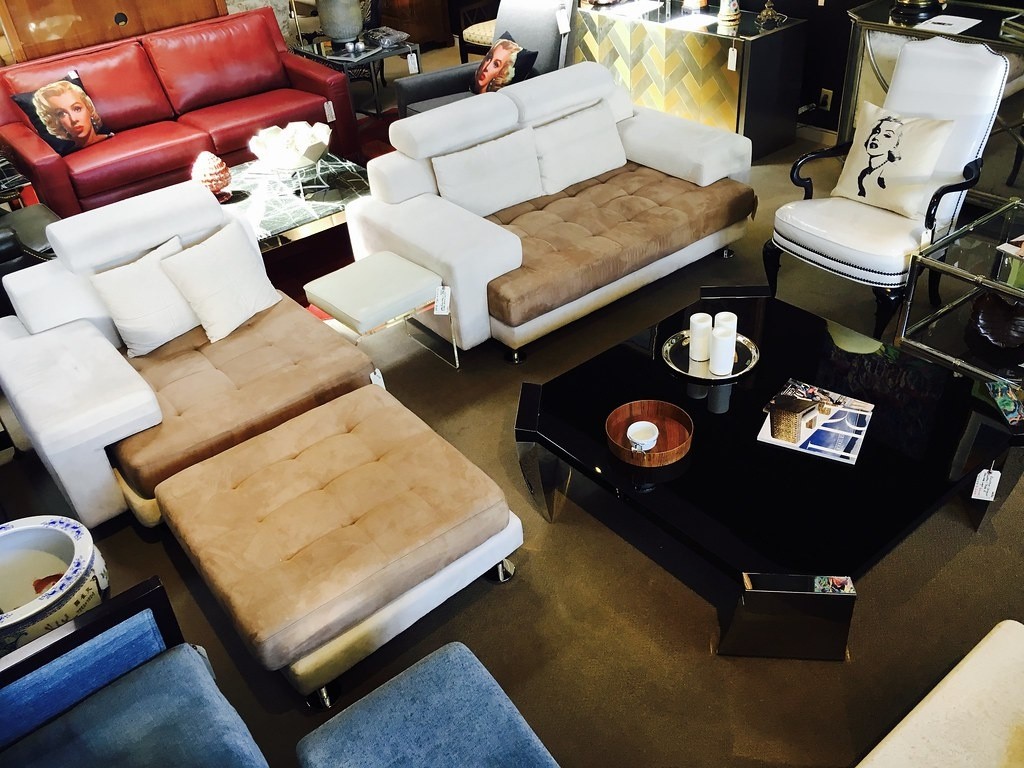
[393,61,540,119]
[345,60,759,365]
[0,180,375,530]
[0,5,359,219]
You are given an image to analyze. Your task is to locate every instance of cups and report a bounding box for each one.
[345,42,364,52]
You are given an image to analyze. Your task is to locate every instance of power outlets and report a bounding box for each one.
[819,88,833,111]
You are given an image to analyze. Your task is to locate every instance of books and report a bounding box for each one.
[326,43,383,63]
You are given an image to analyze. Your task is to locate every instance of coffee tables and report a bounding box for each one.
[513,285,1024,661]
[219,152,371,254]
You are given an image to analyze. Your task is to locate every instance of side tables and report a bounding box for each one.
[894,197,1024,387]
[294,37,423,120]
[303,249,459,370]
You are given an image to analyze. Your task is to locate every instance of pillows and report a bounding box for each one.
[91,235,200,358]
[431,126,545,218]
[533,99,627,196]
[160,219,283,343]
[469,32,538,95]
[9,70,115,156]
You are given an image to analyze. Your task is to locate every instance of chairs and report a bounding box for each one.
[458,0,501,64]
[0,575,268,768]
[0,203,62,318]
[763,36,1010,341]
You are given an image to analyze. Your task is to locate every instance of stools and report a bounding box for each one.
[154,384,524,711]
[295,641,561,768]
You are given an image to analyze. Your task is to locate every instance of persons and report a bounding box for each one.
[31,80,112,148]
[764,377,845,412]
[474,38,524,94]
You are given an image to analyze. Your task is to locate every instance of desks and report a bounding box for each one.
[835,0,1024,186]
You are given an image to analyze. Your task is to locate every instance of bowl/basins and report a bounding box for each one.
[250,121,332,170]
[627,420,659,452]
